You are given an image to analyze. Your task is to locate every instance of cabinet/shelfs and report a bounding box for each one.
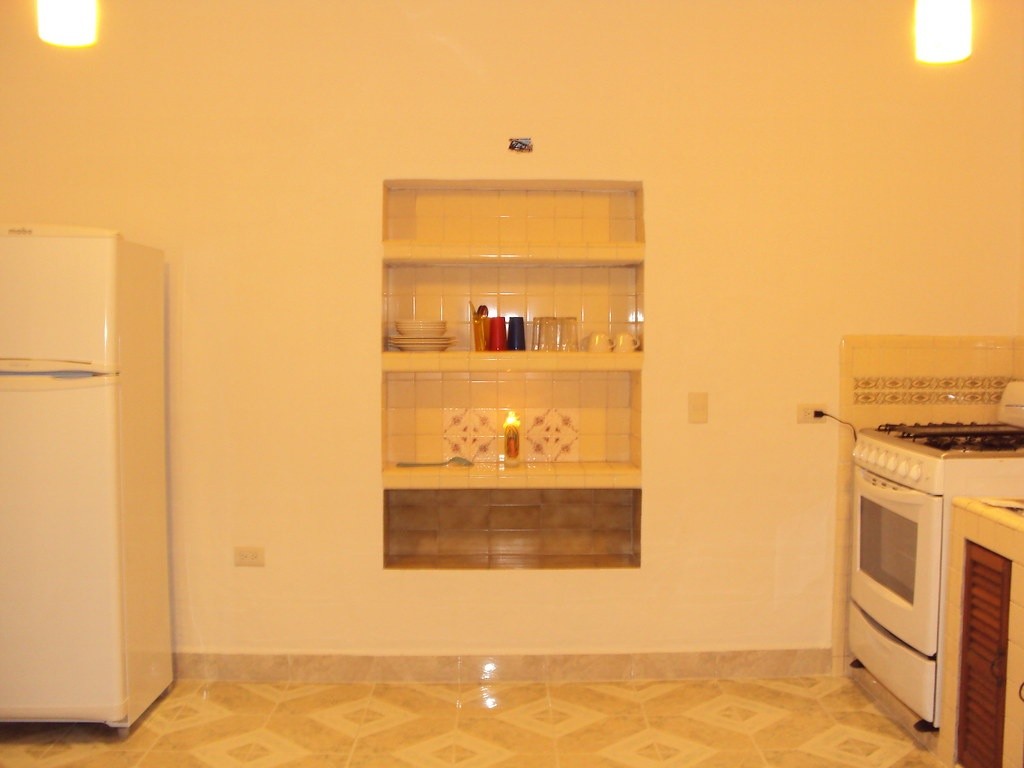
[382,181,644,571]
[939,495,1023,768]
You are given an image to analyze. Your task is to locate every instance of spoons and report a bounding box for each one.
[398,457,473,466]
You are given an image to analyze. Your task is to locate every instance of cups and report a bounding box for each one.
[531,315,579,352]
[489,317,507,350]
[472,318,489,351]
[613,333,640,352]
[507,317,526,350]
[588,333,616,351]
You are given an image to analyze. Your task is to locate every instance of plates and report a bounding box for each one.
[387,320,453,351]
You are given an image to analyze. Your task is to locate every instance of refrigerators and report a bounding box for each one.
[2,223,175,733]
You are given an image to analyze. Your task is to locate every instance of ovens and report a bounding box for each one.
[848,466,1023,733]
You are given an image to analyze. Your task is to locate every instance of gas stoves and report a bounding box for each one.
[855,422,1023,496]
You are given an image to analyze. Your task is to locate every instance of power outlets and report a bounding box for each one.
[798,404,827,424]
[235,547,264,567]
